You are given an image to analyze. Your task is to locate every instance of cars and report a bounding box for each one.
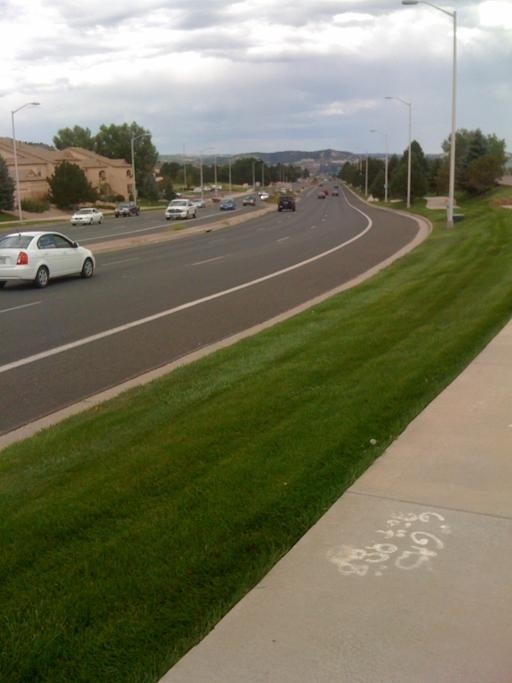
[191,197,207,208]
[164,198,198,219]
[315,173,340,199]
[257,187,288,200]
[1,229,99,290]
[219,197,237,210]
[69,207,105,225]
[242,194,256,206]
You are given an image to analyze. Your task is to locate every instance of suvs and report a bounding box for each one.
[113,200,141,217]
[277,194,297,212]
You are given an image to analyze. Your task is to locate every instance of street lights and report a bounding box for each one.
[130,132,152,203]
[198,146,271,200]
[11,99,42,220]
[383,93,414,208]
[400,0,459,226]
[369,128,391,202]
